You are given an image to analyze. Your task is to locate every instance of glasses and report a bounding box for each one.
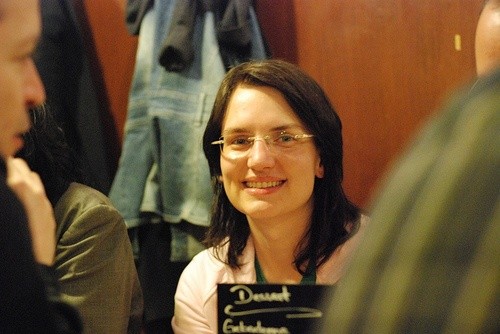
[210,130,314,161]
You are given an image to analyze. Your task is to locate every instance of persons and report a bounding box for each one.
[171,58,373,334]
[0,0,84,334]
[318,0,500,334]
[106,0,267,263]
[12,101,145,334]
